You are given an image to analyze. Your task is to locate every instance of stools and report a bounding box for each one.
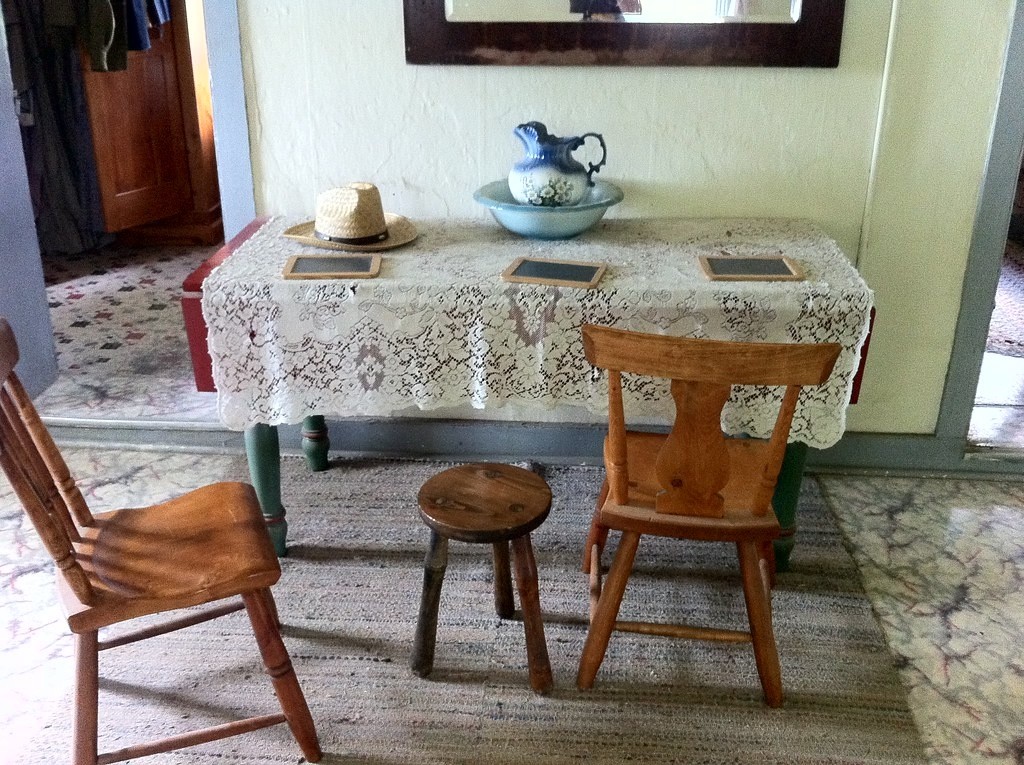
[410,464,557,694]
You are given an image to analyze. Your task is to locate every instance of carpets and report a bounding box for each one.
[22,453,929,765]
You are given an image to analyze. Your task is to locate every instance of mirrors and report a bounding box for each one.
[403,0,846,68]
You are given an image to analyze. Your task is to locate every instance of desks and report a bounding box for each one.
[179,217,875,568]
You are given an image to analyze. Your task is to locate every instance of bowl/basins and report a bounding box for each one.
[473,177,624,238]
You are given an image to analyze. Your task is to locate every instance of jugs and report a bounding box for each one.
[509,122,608,207]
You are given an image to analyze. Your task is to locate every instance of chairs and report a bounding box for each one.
[0,319,319,764]
[579,323,844,705]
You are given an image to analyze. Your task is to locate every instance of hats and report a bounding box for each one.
[283,182,418,251]
